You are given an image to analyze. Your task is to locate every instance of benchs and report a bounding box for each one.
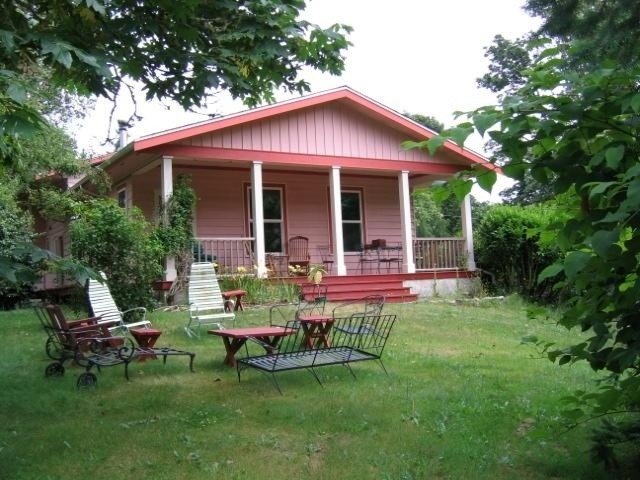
[235,315,397,395]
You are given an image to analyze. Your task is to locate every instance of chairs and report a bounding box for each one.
[32,270,197,394]
[240,231,278,276]
[184,261,386,355]
[286,235,335,277]
